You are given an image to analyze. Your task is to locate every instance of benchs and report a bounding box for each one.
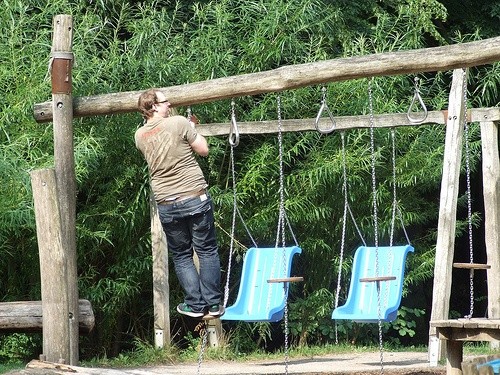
[429,319,500,375]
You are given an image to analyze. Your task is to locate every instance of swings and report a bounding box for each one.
[217,89,304,323]
[331,127,414,325]
[428,66,500,342]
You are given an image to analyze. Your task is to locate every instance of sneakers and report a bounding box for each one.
[209,305,225,316]
[177,303,204,317]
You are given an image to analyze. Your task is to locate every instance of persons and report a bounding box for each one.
[134,88,225,318]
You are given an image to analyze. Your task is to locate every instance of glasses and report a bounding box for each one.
[158,99,168,103]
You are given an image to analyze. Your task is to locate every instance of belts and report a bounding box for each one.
[160,189,204,205]
